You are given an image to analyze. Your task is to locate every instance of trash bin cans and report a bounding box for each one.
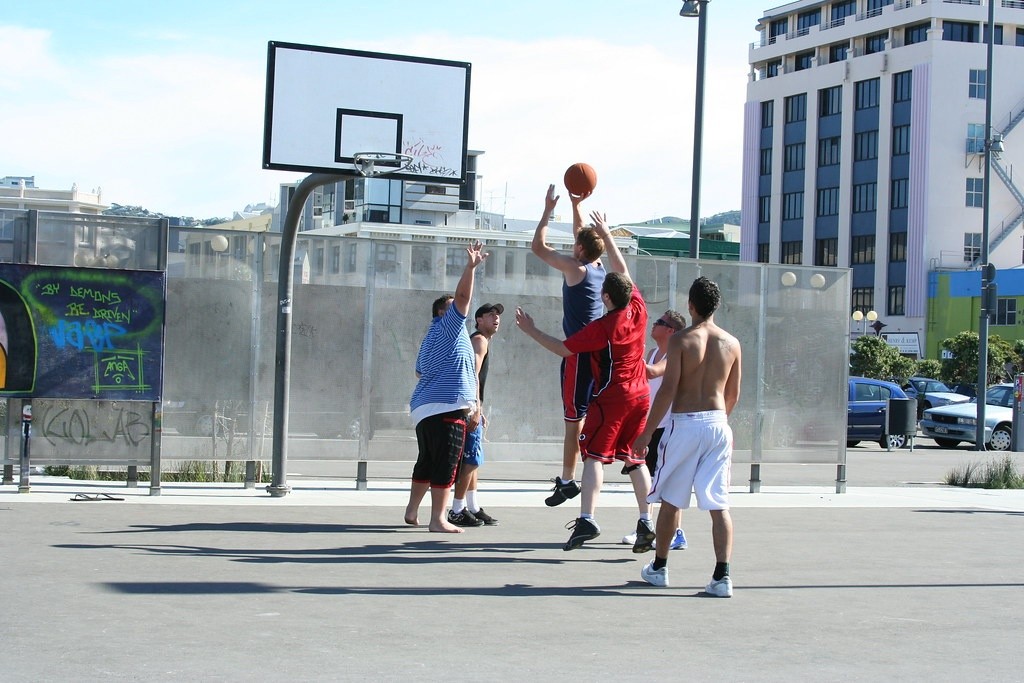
[888,398,917,436]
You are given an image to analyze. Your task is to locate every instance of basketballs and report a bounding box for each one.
[564,163,597,196]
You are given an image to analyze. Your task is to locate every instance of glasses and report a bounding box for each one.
[656,318,676,334]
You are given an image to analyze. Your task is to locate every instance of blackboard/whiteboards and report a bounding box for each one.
[0,263,166,402]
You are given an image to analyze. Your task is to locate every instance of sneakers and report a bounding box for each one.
[641,559,669,587]
[705,576,733,597]
[632,517,656,553]
[651,528,687,550]
[563,517,601,551]
[545,476,581,507]
[470,507,499,525]
[622,530,638,544]
[447,507,484,527]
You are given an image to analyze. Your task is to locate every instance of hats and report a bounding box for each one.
[475,303,504,319]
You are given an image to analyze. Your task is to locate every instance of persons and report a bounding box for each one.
[621,311,687,549]
[404,241,490,531]
[532,182,609,507]
[515,211,656,553]
[448,303,505,526]
[632,278,742,598]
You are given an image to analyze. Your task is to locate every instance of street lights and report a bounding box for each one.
[852,310,878,335]
[679,0,713,259]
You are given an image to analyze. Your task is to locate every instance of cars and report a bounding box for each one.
[920,381,1014,451]
[883,376,971,422]
[846,376,910,449]
